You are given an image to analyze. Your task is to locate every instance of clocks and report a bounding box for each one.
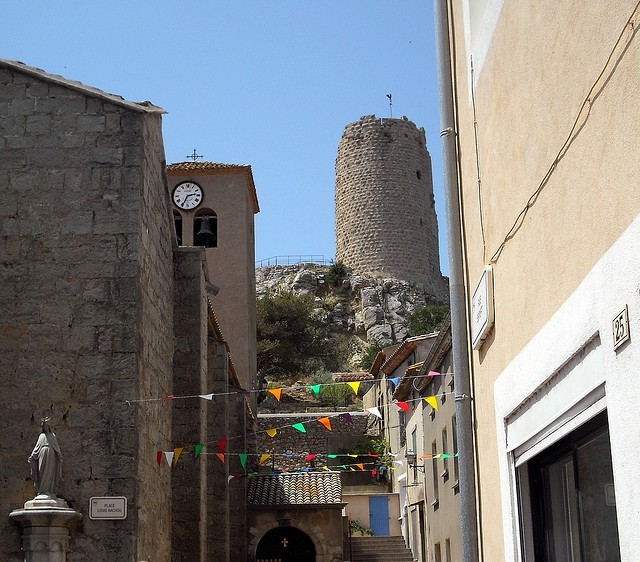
[171,180,204,212]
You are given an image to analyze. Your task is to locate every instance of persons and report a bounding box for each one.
[28,416,63,501]
